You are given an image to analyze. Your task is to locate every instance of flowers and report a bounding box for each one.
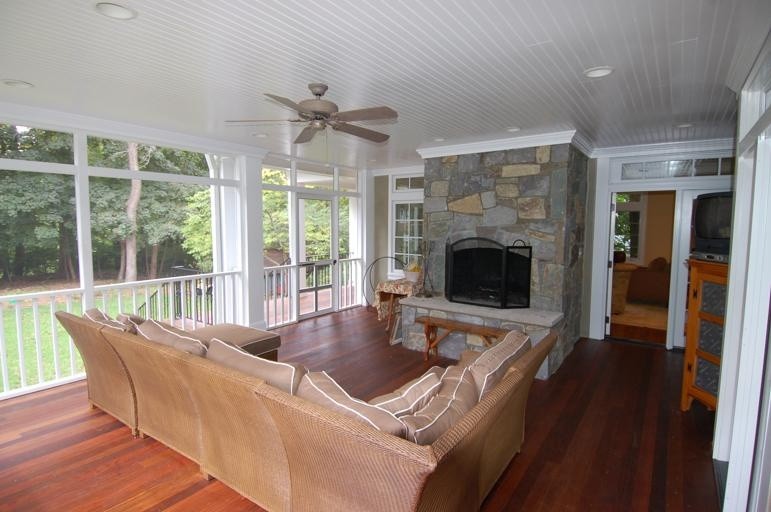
[407,264,421,272]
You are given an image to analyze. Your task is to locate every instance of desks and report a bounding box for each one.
[378,277,422,333]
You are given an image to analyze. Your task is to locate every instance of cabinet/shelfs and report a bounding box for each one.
[679,259,728,411]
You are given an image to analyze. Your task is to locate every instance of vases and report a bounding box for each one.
[407,272,420,282]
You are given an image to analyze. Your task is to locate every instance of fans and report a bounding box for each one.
[223,83,398,152]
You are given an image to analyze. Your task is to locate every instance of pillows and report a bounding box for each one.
[648,258,665,271]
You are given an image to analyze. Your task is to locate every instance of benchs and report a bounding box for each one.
[415,316,509,366]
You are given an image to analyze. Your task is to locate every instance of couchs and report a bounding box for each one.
[626,266,669,307]
[55,304,556,512]
